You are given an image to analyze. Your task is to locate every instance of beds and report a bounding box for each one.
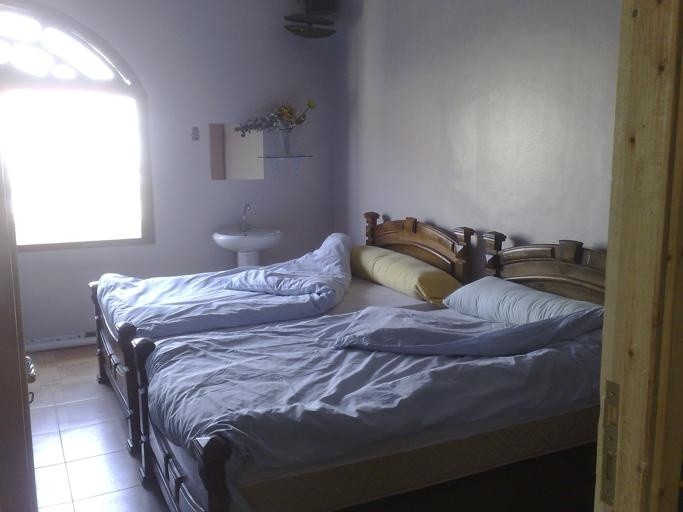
[86,208,475,456]
[126,229,606,512]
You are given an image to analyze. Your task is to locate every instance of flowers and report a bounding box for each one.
[236,95,317,137]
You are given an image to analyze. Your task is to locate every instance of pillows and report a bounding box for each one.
[349,245,464,308]
[448,277,600,329]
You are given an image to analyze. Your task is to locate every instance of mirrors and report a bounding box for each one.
[208,122,265,180]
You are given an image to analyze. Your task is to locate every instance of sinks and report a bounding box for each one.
[212,226,286,251]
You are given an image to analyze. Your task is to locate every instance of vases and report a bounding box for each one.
[277,130,291,156]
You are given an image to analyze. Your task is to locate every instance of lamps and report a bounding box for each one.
[282,1,335,39]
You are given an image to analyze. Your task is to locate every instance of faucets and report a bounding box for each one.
[244,202,252,218]
